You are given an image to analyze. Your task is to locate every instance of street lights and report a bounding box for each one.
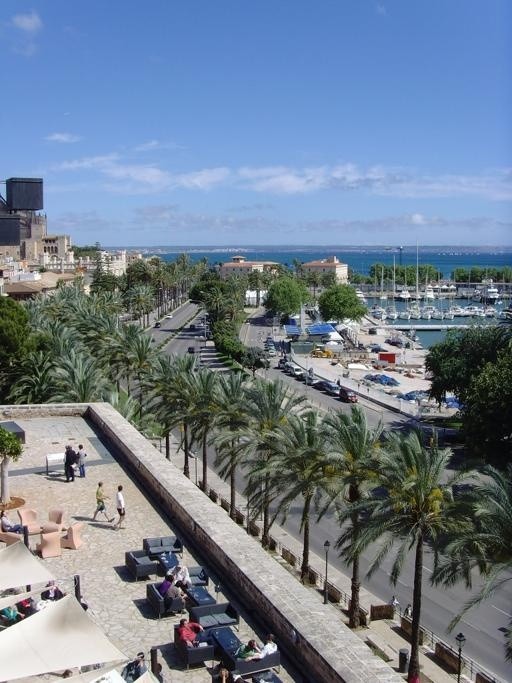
[323,540,331,604]
[455,633,466,683]
[189,299,207,349]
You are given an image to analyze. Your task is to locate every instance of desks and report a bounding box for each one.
[46,452,66,475]
[1,419,26,444]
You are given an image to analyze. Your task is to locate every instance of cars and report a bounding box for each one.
[265,335,357,403]
[154,313,173,328]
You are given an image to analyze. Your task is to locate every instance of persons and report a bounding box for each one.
[77,444,87,478]
[234,639,261,660]
[244,633,278,662]
[177,618,215,646]
[159,565,193,614]
[216,668,234,683]
[114,485,125,530]
[91,481,115,522]
[388,596,399,612]
[131,652,147,681]
[404,604,413,618]
[1,511,30,547]
[0,580,69,625]
[62,445,76,482]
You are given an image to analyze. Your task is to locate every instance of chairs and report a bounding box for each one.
[124,533,284,681]
[0,503,85,559]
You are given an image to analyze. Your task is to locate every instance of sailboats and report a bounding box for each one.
[369,238,512,324]
